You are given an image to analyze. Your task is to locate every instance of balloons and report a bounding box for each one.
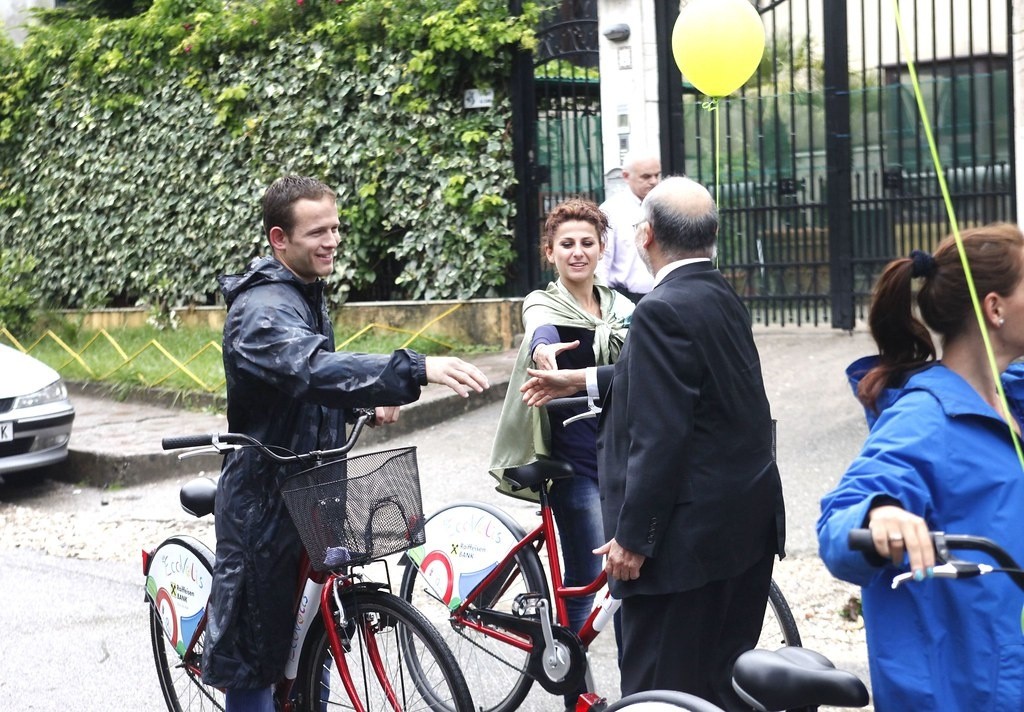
[671,0,766,99]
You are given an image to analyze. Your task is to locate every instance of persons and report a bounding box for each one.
[596,143,662,307]
[814,218,1024,711]
[520,173,788,712]
[196,173,492,712]
[485,195,638,712]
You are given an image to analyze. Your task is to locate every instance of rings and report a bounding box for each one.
[888,532,904,542]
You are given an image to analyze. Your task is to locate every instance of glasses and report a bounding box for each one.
[632,220,648,233]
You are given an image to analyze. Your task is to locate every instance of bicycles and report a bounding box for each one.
[397,454,804,712]
[139,409,476,712]
[577,531,1024,712]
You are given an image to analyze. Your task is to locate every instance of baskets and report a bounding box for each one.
[278,446,426,572]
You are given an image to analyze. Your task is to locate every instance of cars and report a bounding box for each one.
[0,343,76,474]
